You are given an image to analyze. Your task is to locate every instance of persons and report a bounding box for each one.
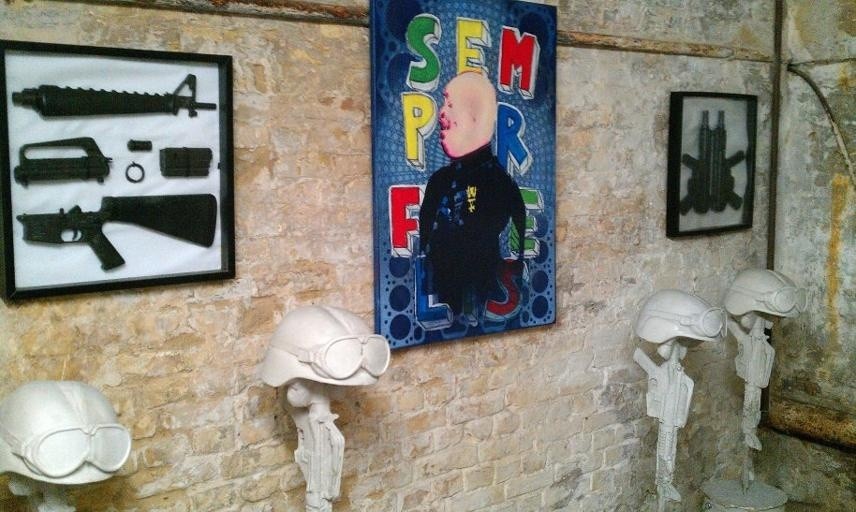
[418,70,529,323]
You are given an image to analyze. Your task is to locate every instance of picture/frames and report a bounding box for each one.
[666,91,760,242]
[0,36,236,304]
